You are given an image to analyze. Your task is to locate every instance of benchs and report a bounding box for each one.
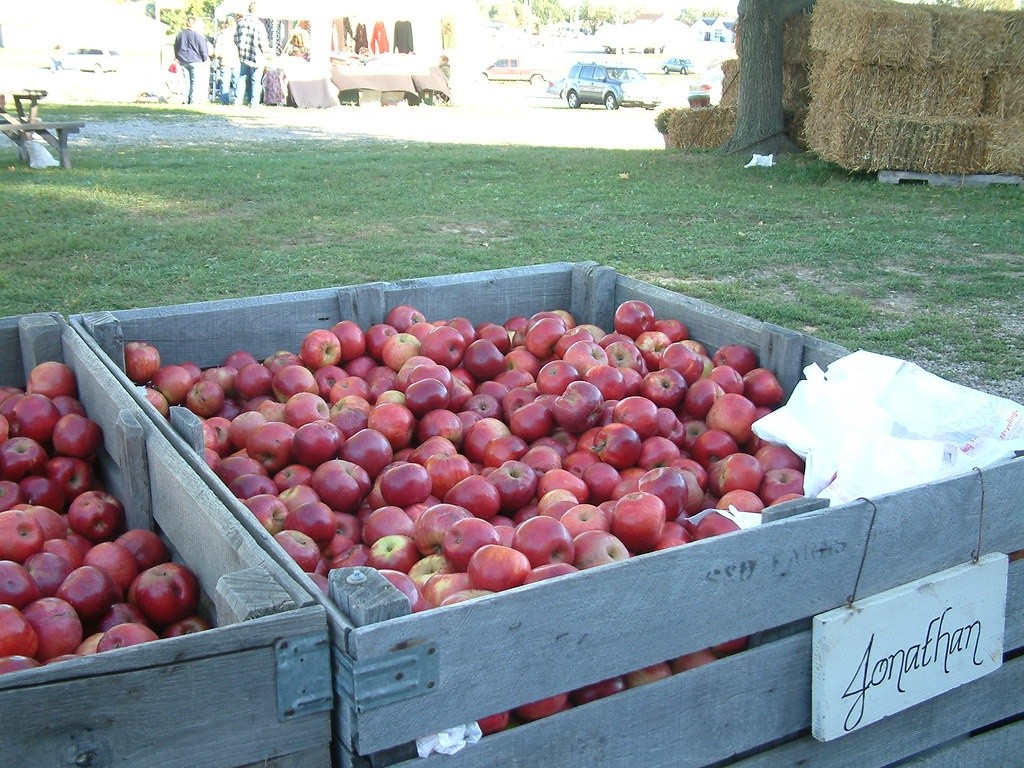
[0,121,85,173]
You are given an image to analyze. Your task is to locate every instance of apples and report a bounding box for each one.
[1,299,808,737]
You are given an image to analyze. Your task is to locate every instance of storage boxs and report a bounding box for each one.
[63,257,1024,768]
[0,310,334,767]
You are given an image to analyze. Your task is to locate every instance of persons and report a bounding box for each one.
[174,15,209,105]
[217,16,239,105]
[234,2,268,108]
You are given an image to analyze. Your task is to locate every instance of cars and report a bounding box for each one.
[482,58,546,85]
[73,49,121,74]
[661,56,696,75]
[546,76,567,101]
[565,60,662,109]
